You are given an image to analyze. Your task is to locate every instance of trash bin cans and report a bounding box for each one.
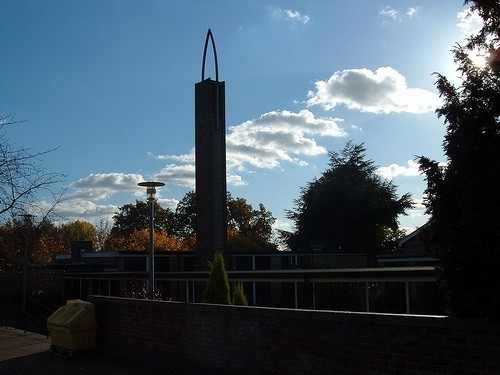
[45,299,96,359]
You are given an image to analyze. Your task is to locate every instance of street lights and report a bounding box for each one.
[18,214,37,333]
[138,182,166,301]
